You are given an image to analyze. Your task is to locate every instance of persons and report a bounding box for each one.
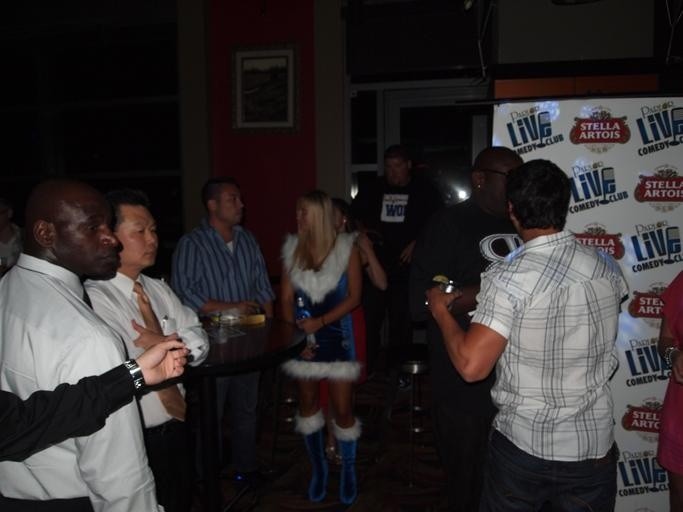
[656,270,683,512]
[280,145,439,506]
[0,189,25,277]
[408,148,524,512]
[83,188,210,512]
[0,341,188,462]
[426,159,629,512]
[0,179,166,512]
[171,176,277,507]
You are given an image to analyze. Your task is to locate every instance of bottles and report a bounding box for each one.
[294,297,316,347]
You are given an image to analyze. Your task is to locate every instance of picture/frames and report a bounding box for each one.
[230,42,298,133]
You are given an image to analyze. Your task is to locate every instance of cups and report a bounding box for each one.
[424,282,456,313]
[208,307,239,343]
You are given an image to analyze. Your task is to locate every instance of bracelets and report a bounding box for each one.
[663,346,680,368]
[124,359,145,392]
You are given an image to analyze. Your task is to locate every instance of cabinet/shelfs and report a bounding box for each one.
[0,8,179,248]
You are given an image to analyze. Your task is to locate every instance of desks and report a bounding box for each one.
[185,317,307,512]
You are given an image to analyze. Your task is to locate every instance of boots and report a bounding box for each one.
[331,416,364,508]
[296,409,330,504]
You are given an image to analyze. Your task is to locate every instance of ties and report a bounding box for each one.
[133,282,188,424]
